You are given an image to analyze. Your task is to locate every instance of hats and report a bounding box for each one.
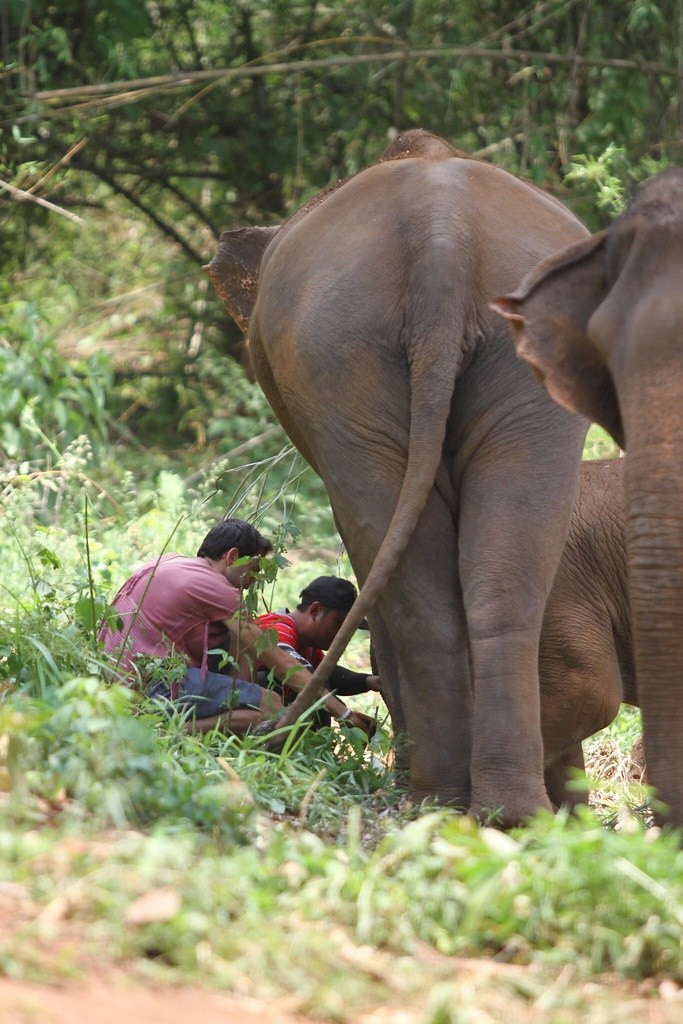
[300,575,369,631]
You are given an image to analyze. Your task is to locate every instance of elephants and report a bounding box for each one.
[539,458,626,812]
[198,128,594,826]
[486,170,683,830]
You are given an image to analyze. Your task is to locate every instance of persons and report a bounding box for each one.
[248,575,383,738]
[96,518,369,740]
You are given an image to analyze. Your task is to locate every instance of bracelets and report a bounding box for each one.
[334,708,351,722]
